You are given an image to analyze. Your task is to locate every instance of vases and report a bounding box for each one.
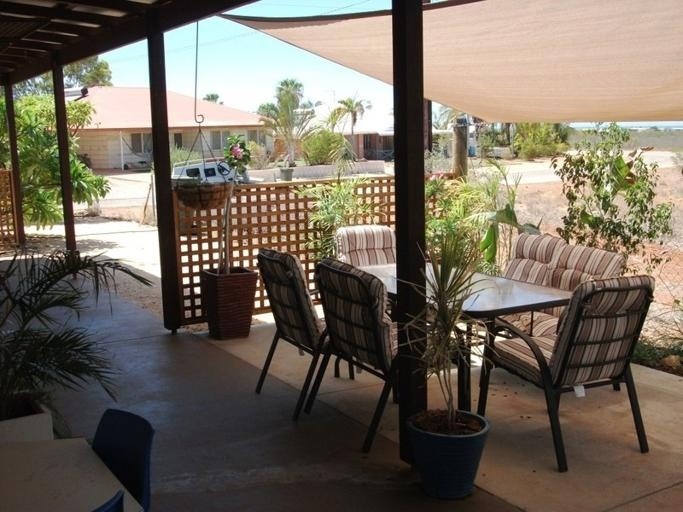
[200,264,259,342]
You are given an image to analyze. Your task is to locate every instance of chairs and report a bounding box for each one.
[92,407,156,510]
[89,487,125,511]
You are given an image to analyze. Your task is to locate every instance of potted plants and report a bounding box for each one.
[388,211,496,501]
[174,172,234,211]
[0,243,124,441]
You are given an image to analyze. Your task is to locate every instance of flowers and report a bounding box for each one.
[216,131,251,273]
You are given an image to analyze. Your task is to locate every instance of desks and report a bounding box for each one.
[1,433,147,512]
